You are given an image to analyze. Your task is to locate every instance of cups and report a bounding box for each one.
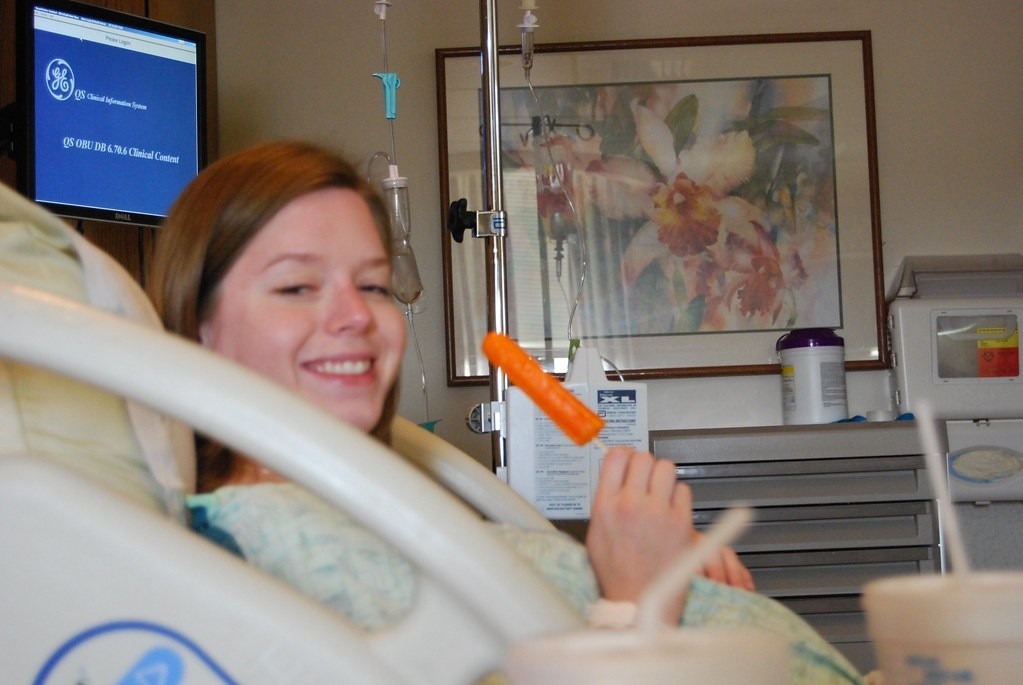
[860,571,1022,683]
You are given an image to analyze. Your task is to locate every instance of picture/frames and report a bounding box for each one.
[434,28,887,385]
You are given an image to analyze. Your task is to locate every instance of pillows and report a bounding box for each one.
[0,181,198,534]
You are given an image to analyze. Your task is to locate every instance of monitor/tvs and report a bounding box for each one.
[14,0,209,229]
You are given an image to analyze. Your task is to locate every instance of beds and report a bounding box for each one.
[0,184,869,685]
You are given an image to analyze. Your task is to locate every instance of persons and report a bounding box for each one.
[146,141,756,684]
